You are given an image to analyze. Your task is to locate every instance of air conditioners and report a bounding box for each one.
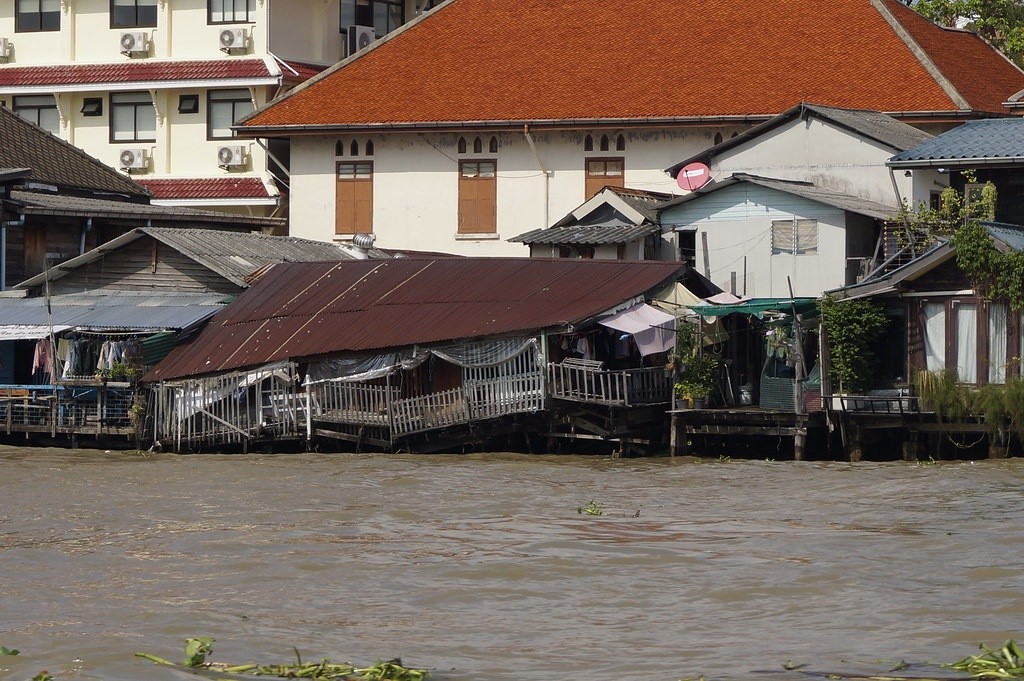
[347,24,375,58]
[118,149,149,173]
[119,31,150,57]
[0,37,13,57]
[218,146,248,171]
[219,28,248,55]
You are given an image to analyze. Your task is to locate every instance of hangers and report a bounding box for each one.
[34,331,140,349]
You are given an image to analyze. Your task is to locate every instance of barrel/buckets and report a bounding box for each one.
[738,385,753,405]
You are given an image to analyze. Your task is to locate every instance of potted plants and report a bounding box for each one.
[665,323,722,410]
[95,361,141,383]
[758,328,800,411]
[824,290,885,410]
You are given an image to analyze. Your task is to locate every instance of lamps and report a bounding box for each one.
[904,168,913,178]
